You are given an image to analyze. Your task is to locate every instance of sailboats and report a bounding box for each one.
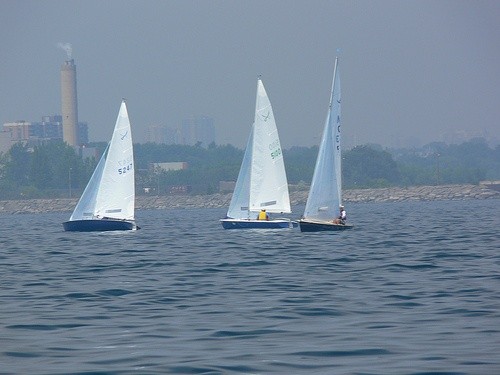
[60,95,144,232]
[218,75,299,230]
[297,56,356,231]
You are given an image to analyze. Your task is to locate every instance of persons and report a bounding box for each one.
[257,208,270,221]
[336,206,346,224]
